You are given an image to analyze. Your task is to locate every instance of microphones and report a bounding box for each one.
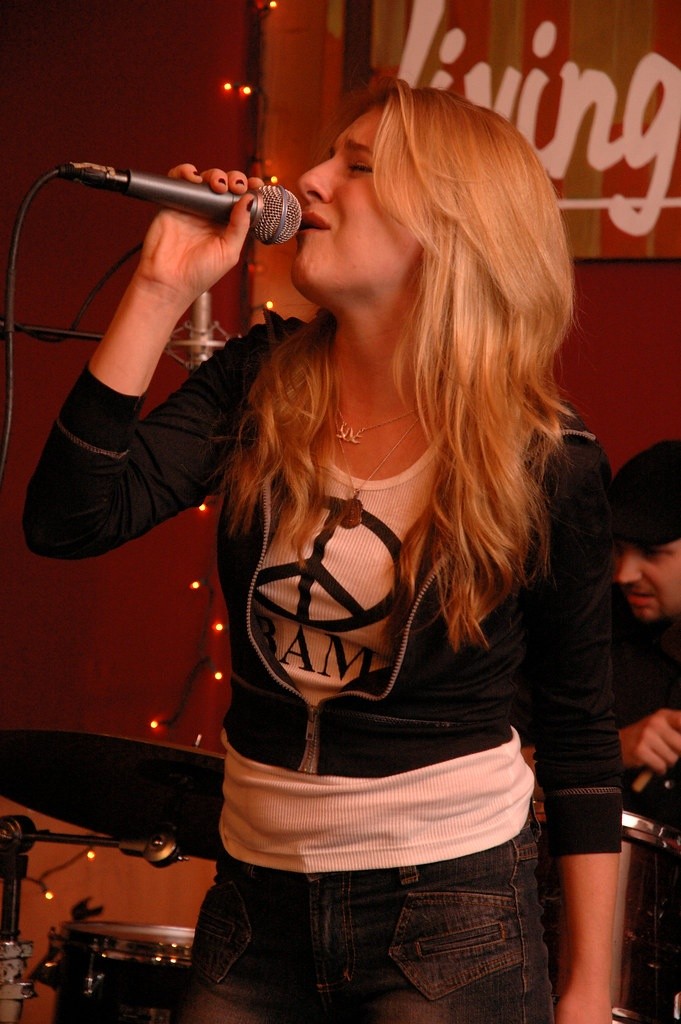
[55,161,301,246]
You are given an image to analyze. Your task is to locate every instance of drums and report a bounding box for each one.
[54,918,195,1024]
[532,796,681,1024]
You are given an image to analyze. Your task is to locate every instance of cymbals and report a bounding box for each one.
[0,726,225,861]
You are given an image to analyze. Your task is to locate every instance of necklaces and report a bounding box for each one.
[331,389,441,529]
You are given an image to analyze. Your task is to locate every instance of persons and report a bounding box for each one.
[21,79,623,1024]
[510,440,681,829]
